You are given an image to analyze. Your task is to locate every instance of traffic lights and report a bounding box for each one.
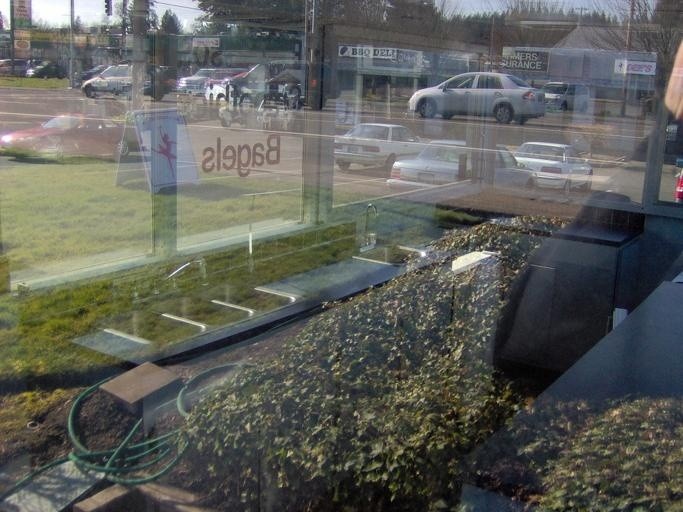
[104,0,112,16]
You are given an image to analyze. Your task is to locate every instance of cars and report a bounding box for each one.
[333,122,422,173]
[2,112,144,163]
[513,141,592,194]
[409,70,545,127]
[387,140,535,200]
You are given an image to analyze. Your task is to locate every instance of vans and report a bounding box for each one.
[536,82,591,114]
